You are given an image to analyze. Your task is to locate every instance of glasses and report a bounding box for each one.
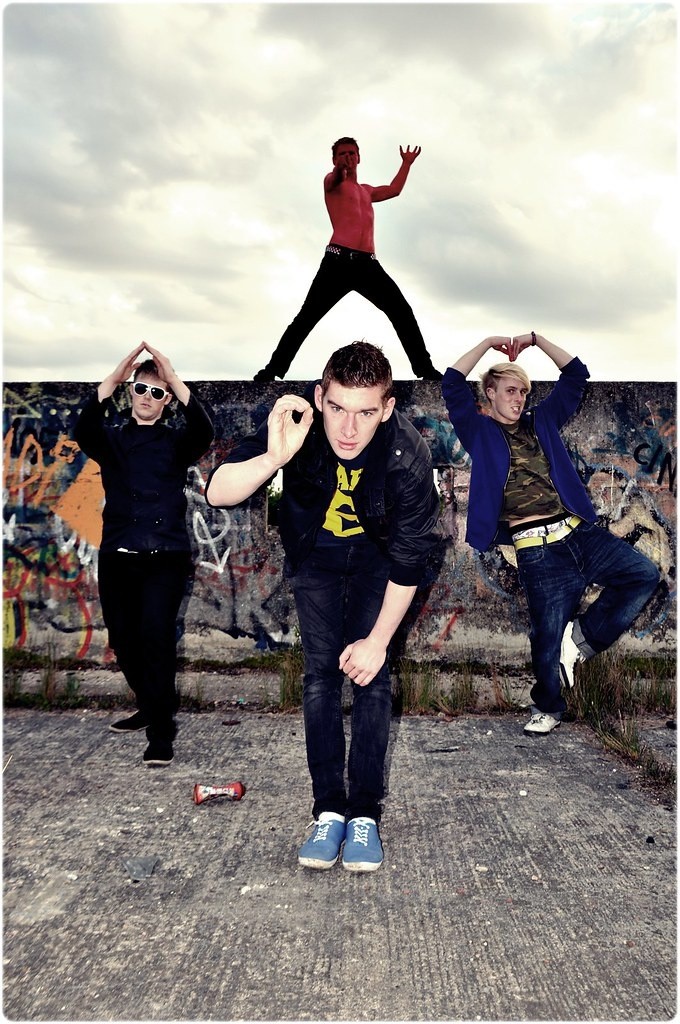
[132,382,169,402]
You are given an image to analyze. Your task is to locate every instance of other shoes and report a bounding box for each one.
[423,368,444,380]
[252,364,275,382]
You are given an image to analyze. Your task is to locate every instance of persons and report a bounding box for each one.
[252,136,445,380]
[204,340,444,871]
[75,341,214,767]
[441,332,662,736]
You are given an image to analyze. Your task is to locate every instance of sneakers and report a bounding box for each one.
[297,811,383,872]
[108,710,174,765]
[523,714,561,738]
[558,620,581,691]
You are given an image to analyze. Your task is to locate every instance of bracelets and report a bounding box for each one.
[531,331,537,346]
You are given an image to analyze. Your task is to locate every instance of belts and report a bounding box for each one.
[325,244,376,261]
[513,515,582,551]
